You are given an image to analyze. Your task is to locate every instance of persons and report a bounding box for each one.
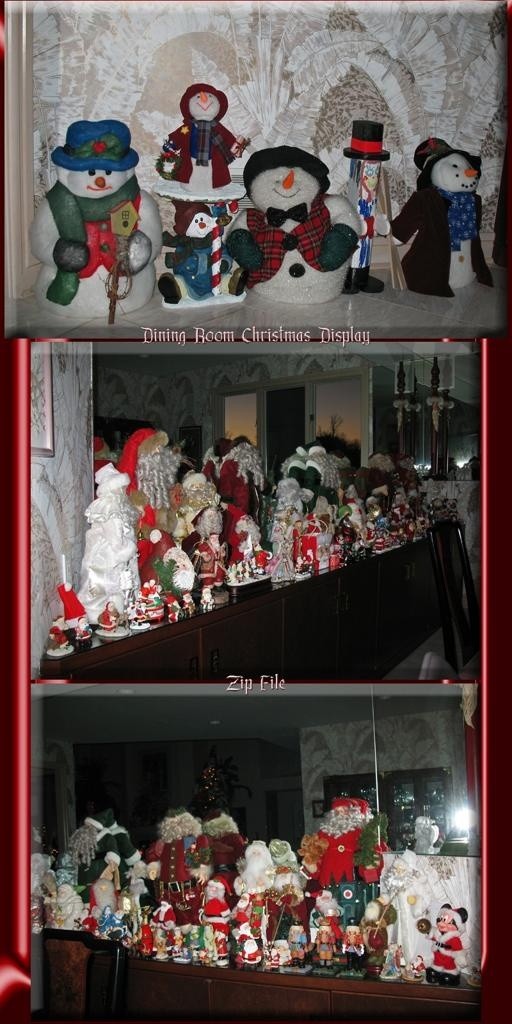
[157,195,250,308]
[227,146,361,305]
[392,137,495,297]
[342,119,394,294]
[30,785,484,988]
[44,423,460,658]
[154,84,250,202]
[28,121,162,326]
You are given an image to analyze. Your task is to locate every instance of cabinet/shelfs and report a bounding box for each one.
[41,527,459,684]
[128,947,482,1022]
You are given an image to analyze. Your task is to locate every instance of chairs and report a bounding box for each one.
[34,928,128,1022]
